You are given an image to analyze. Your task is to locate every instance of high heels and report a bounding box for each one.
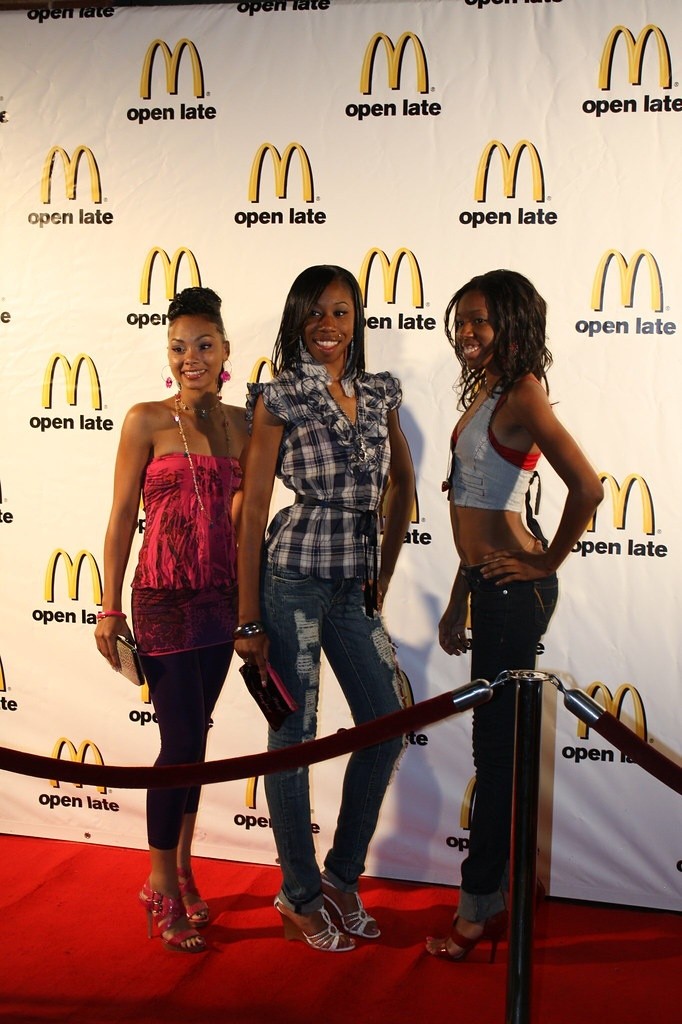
[171,865,208,929]
[317,872,382,941]
[137,878,207,954]
[272,893,356,954]
[425,909,507,966]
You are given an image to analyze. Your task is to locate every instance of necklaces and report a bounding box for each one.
[323,374,368,462]
[174,389,233,530]
[442,376,496,494]
[176,391,222,420]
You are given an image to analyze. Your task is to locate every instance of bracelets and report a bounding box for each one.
[95,611,128,621]
[233,622,265,641]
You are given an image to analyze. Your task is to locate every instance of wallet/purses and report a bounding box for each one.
[114,637,145,689]
[239,658,300,734]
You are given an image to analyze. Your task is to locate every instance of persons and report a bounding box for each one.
[96,287,255,953]
[425,270,605,968]
[233,264,417,953]
